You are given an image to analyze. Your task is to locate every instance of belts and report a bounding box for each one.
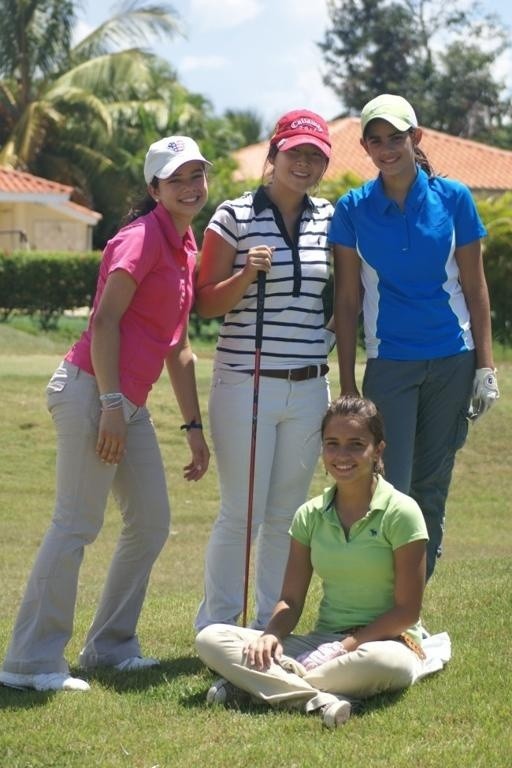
[240,365,329,381]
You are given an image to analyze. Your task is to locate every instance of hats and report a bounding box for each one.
[269,110,332,159]
[359,94,418,142]
[144,136,214,186]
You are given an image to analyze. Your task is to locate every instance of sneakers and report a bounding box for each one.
[317,696,364,728]
[110,657,160,670]
[206,677,250,708]
[0,670,90,691]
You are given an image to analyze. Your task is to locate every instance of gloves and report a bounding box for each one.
[466,368,498,419]
[296,641,347,671]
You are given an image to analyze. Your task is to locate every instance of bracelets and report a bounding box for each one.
[98,393,125,411]
[180,417,202,432]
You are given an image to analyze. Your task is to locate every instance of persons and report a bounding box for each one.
[194,395,429,730]
[193,109,334,639]
[1,135,211,693]
[327,94,500,638]
